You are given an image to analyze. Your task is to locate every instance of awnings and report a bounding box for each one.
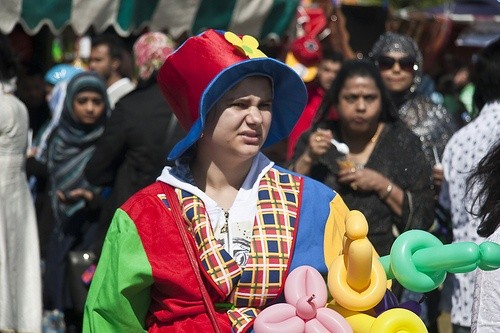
[0,0,300,46]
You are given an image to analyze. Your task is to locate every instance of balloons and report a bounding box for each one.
[253,210,500,333]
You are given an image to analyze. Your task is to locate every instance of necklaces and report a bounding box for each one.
[370,131,378,143]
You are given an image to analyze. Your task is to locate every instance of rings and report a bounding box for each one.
[316,136,321,141]
[352,168,356,174]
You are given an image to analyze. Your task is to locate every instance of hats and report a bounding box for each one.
[135,31,173,79]
[157,28,308,162]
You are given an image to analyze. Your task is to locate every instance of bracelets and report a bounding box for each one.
[381,182,393,201]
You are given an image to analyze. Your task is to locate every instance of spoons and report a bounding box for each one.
[433,146,441,167]
[330,138,350,155]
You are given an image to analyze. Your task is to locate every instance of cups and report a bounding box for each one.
[434,165,443,185]
[336,155,356,170]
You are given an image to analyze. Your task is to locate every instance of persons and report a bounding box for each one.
[0,29,436,333]
[438,39,500,333]
[372,32,461,188]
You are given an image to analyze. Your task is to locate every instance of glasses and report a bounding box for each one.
[379,53,414,73]
